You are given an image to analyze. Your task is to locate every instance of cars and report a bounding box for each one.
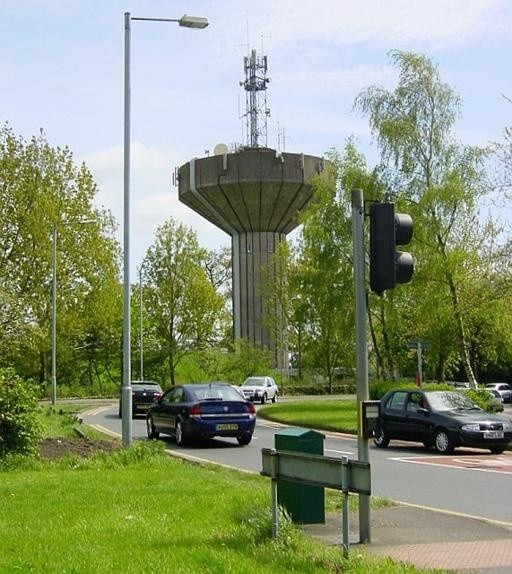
[447,380,512,412]
[373,388,511,456]
[145,382,257,448]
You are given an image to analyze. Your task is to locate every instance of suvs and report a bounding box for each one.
[240,375,278,405]
[118,381,165,419]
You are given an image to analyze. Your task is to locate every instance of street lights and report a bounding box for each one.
[122,11,211,446]
[50,217,98,405]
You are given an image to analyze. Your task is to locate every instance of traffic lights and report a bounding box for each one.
[367,201,416,294]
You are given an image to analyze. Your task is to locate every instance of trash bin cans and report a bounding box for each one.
[273,426,325,526]
